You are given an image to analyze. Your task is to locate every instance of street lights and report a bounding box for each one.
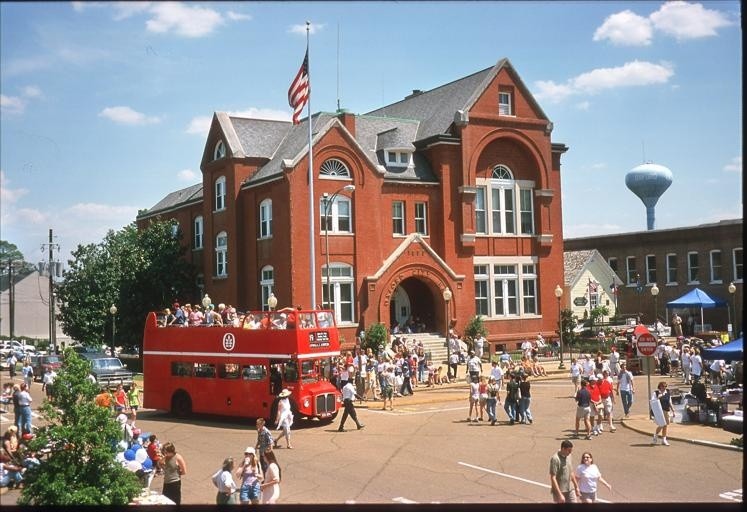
[321,183,355,309]
[728,281,738,340]
[442,286,455,383]
[108,303,117,355]
[651,281,659,333]
[554,282,565,370]
[201,294,212,311]
[267,292,278,311]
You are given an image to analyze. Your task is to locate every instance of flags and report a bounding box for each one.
[590,281,596,293]
[288,49,308,126]
[635,280,644,293]
[610,283,618,294]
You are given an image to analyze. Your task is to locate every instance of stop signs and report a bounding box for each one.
[636,335,658,357]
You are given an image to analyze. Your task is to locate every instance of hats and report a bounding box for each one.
[471,350,475,354]
[244,447,256,457]
[348,378,354,380]
[116,413,128,424]
[589,375,599,381]
[279,389,292,398]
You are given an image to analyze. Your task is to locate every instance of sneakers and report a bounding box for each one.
[652,434,670,446]
[467,418,533,425]
[570,427,616,440]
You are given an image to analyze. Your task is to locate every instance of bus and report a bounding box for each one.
[141,310,343,430]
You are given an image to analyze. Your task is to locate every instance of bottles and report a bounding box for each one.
[245,456,250,465]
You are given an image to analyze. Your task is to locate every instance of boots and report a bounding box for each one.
[338,425,347,432]
[357,424,364,430]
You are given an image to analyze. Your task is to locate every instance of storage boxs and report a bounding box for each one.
[722,415,743,434]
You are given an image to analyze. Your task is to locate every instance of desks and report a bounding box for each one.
[707,391,743,410]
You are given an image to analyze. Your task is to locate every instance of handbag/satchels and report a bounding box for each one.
[466,375,471,384]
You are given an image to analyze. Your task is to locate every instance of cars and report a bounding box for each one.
[618,336,637,350]
[64,346,86,358]
[0,340,38,359]
[575,330,612,343]
[25,353,67,377]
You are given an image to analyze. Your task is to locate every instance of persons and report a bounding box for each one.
[85,370,141,429]
[1,382,42,489]
[176,362,294,397]
[274,389,293,449]
[568,313,746,445]
[550,440,580,503]
[158,302,312,328]
[575,452,612,503]
[42,365,57,407]
[6,350,34,389]
[316,304,545,433]
[106,414,186,504]
[211,418,281,505]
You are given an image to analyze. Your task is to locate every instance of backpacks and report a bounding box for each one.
[489,384,496,397]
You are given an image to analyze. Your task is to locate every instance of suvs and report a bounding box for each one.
[76,352,136,394]
